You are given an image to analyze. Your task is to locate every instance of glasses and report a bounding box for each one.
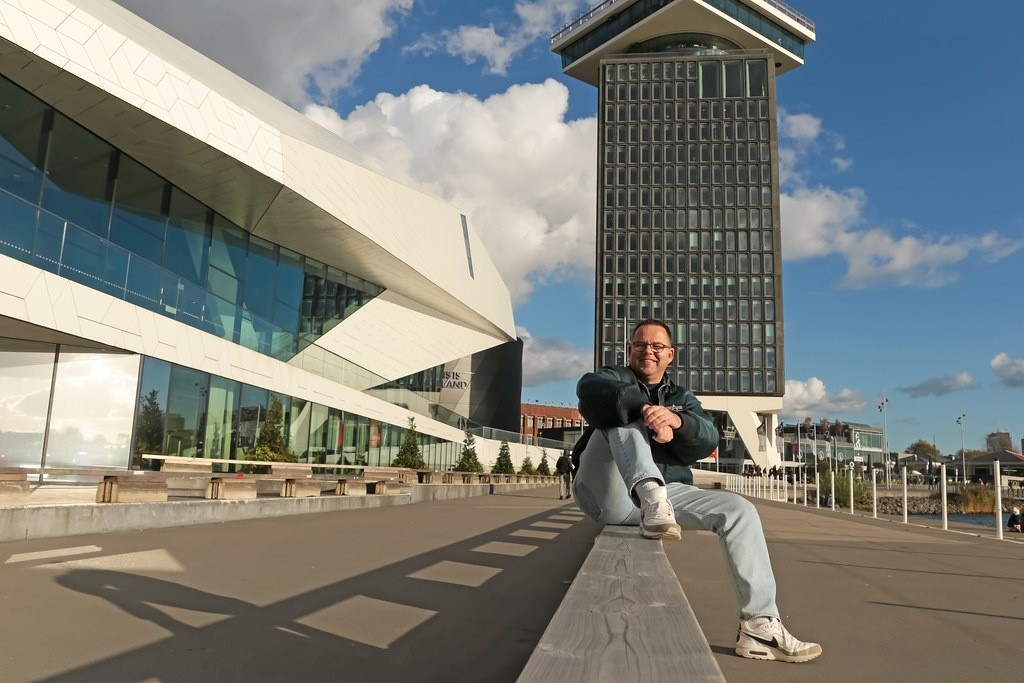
[632,341,670,352]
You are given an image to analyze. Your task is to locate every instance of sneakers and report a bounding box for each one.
[639,497,681,540]
[734,619,822,663]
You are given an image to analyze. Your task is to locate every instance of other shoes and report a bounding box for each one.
[566,496,571,499]
[560,496,564,500]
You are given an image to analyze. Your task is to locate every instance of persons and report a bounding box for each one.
[556,449,573,500]
[572,320,823,663]
[928,477,939,490]
[742,462,809,484]
[1007,507,1021,532]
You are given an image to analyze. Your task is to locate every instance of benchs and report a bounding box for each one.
[515,525,726,683]
[1,453,560,507]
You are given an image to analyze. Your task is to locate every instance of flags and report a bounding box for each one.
[708,449,717,457]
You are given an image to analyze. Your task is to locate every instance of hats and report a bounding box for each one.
[1013,507,1020,515]
